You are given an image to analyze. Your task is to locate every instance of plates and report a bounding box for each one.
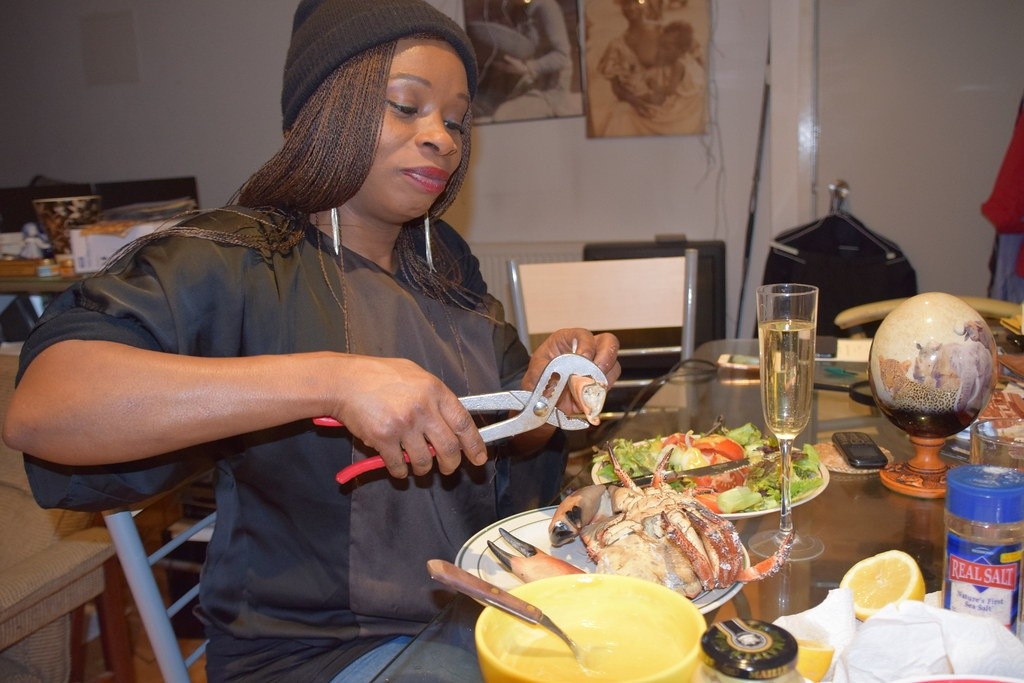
[455,499,751,617]
[596,433,829,520]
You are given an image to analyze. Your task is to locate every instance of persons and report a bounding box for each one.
[0,0,623,682]
[474,0,575,124]
[594,0,706,136]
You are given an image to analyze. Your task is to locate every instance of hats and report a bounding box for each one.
[279,2,478,136]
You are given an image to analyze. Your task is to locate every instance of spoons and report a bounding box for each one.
[426,558,614,674]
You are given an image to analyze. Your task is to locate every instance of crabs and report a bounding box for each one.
[487,441,795,601]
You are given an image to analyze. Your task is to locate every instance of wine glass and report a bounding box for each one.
[747,281,826,562]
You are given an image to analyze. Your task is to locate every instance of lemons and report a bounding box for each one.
[798,549,924,682]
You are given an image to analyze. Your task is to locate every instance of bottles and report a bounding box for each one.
[942,463,1021,645]
[694,616,803,683]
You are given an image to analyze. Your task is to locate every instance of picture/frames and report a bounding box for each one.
[578,1,714,139]
[454,0,589,128]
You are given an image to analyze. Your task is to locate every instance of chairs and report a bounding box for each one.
[1,183,93,234]
[2,341,192,682]
[101,470,219,683]
[96,175,201,214]
[583,240,727,411]
[506,248,699,461]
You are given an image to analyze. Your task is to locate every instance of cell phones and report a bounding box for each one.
[832,430,889,470]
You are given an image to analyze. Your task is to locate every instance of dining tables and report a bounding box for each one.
[0,262,95,293]
[364,335,1022,683]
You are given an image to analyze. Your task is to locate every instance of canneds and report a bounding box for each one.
[695,617,804,683]
[943,466,1022,640]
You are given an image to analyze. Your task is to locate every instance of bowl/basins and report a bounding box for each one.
[472,574,707,681]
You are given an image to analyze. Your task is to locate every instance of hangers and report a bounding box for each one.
[766,180,909,266]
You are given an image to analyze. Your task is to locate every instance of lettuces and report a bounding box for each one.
[590,410,825,514]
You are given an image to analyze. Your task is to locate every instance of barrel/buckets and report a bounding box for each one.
[31,194,101,256]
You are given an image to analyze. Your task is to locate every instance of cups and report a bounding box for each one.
[967,418,1024,477]
